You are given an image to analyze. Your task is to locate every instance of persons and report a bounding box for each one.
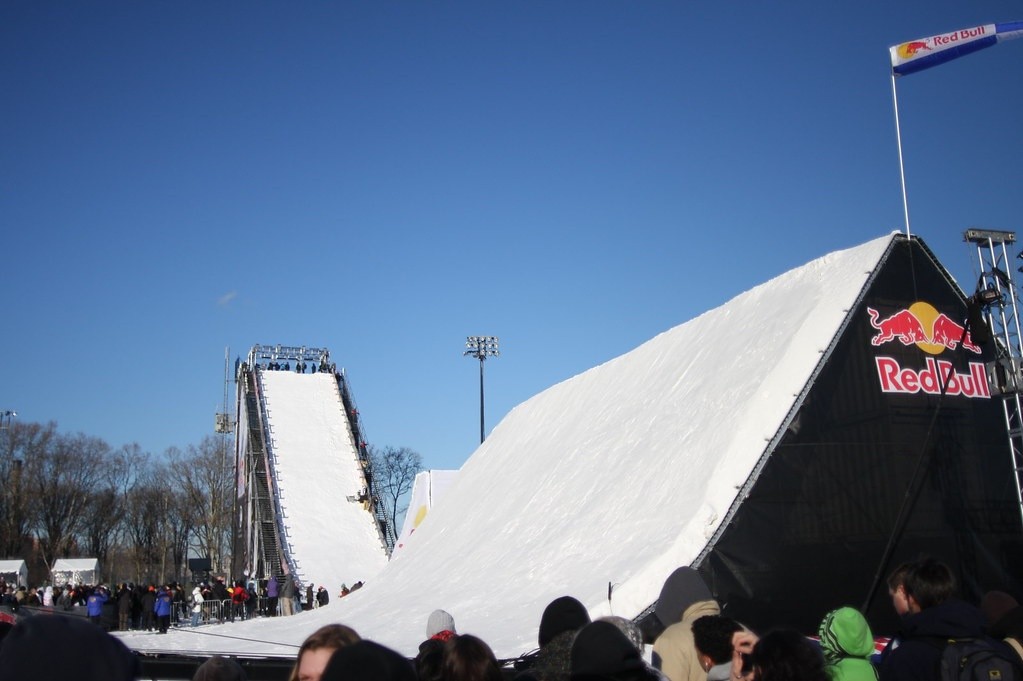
[416,566,1023,681]
[0,612,136,681]
[0,573,365,635]
[290,624,414,681]
[190,656,246,680]
[267,361,316,374]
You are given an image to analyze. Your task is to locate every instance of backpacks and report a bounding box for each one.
[906,635,1023,681]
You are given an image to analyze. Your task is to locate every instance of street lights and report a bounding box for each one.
[463,335,501,445]
